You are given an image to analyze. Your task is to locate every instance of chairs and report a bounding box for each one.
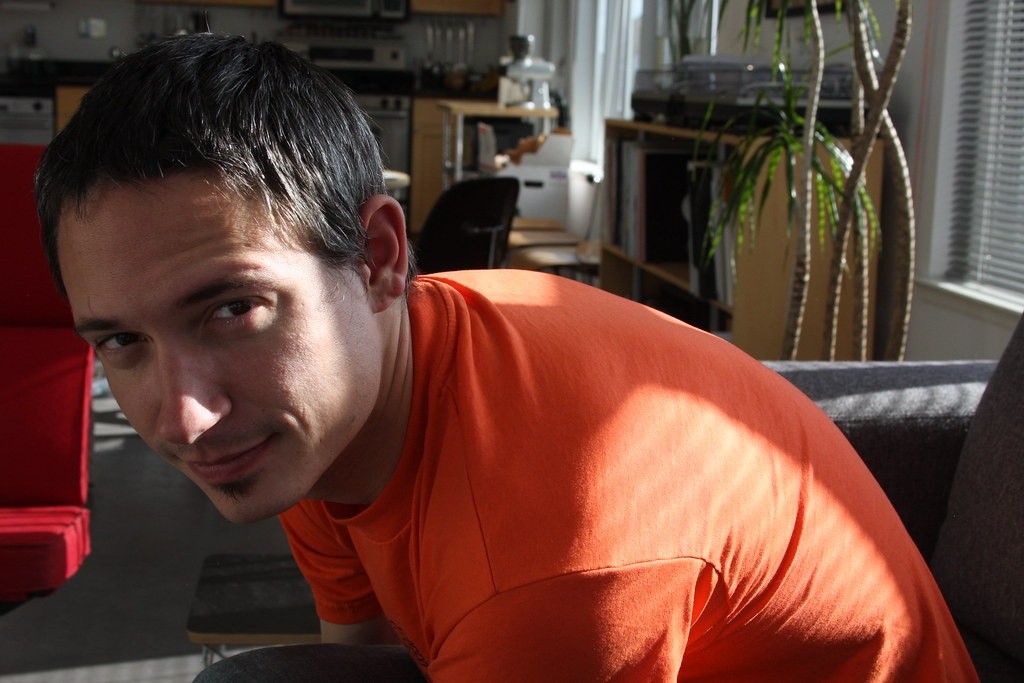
[0,141,93,620]
[418,176,521,277]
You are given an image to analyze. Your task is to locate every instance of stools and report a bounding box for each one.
[188,554,326,666]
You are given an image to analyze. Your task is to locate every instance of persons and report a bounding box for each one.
[35,34,980,683]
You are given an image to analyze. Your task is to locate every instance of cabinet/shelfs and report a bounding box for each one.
[602,113,879,366]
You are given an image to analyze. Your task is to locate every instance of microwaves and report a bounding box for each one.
[274,0,414,22]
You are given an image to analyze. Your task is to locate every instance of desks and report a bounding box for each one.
[405,99,562,232]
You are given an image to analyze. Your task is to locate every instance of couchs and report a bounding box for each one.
[743,318,1024,683]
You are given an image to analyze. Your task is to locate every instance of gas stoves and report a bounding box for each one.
[270,26,415,100]
